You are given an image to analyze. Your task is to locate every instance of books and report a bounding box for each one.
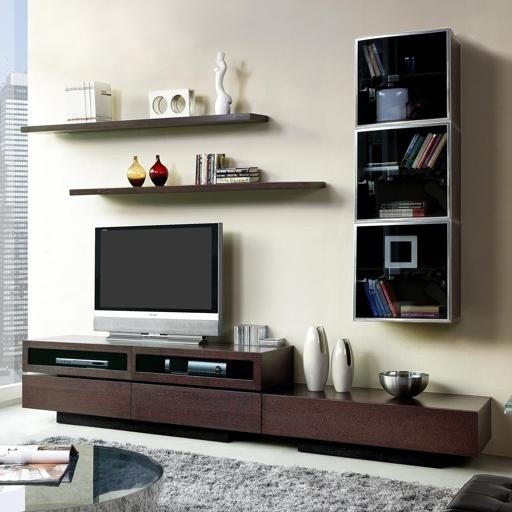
[363,43,386,76]
[379,202,426,217]
[400,307,440,318]
[362,278,397,317]
[401,133,448,168]
[0,442,79,486]
[365,161,400,171]
[194,153,259,184]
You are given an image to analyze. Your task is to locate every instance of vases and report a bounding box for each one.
[149,155,168,187]
[302,324,330,393]
[331,339,354,394]
[126,156,147,187]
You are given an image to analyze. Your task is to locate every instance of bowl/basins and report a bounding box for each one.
[378,371,429,398]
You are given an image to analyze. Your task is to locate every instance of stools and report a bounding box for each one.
[449,469,512,512]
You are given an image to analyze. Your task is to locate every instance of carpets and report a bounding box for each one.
[1,434,456,512]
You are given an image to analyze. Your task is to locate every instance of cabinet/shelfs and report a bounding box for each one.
[20,114,324,196]
[20,339,492,465]
[356,30,458,326]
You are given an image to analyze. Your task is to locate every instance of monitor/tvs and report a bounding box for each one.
[93,223,223,345]
